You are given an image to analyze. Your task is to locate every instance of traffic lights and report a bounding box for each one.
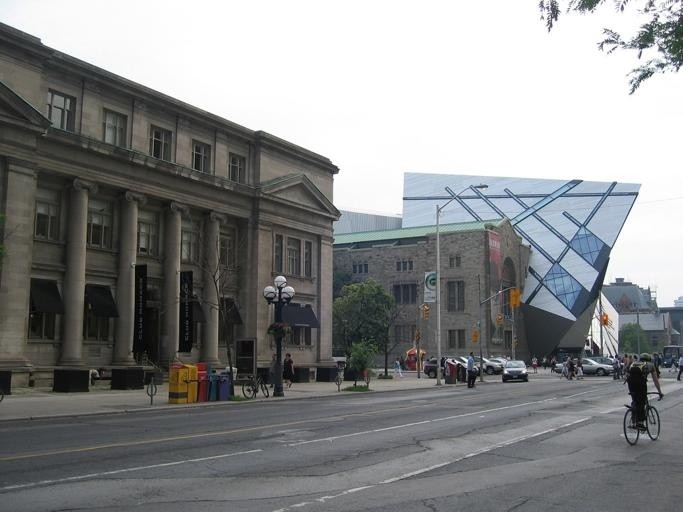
[423,304,430,320]
[415,329,420,340]
[602,314,608,325]
[496,314,503,322]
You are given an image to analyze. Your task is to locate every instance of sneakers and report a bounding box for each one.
[635,420,648,431]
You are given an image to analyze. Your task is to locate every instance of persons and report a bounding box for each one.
[441,356,447,377]
[394,357,402,377]
[268,352,277,388]
[506,355,511,360]
[281,353,294,389]
[624,352,664,431]
[399,355,404,371]
[466,351,476,388]
[530,351,682,383]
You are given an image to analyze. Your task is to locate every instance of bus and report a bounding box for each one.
[663,345,683,368]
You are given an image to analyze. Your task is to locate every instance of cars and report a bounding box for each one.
[423,355,507,378]
[553,356,624,377]
[502,360,528,383]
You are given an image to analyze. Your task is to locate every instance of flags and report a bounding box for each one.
[509,288,521,308]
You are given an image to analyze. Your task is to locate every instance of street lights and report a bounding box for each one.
[262,275,295,397]
[436,184,489,385]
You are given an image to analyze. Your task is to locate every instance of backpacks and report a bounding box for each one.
[626,364,643,393]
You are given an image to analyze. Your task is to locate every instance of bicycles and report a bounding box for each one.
[623,392,662,445]
[242,370,269,399]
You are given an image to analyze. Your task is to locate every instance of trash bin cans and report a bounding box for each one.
[209,374,231,401]
[444,359,466,384]
